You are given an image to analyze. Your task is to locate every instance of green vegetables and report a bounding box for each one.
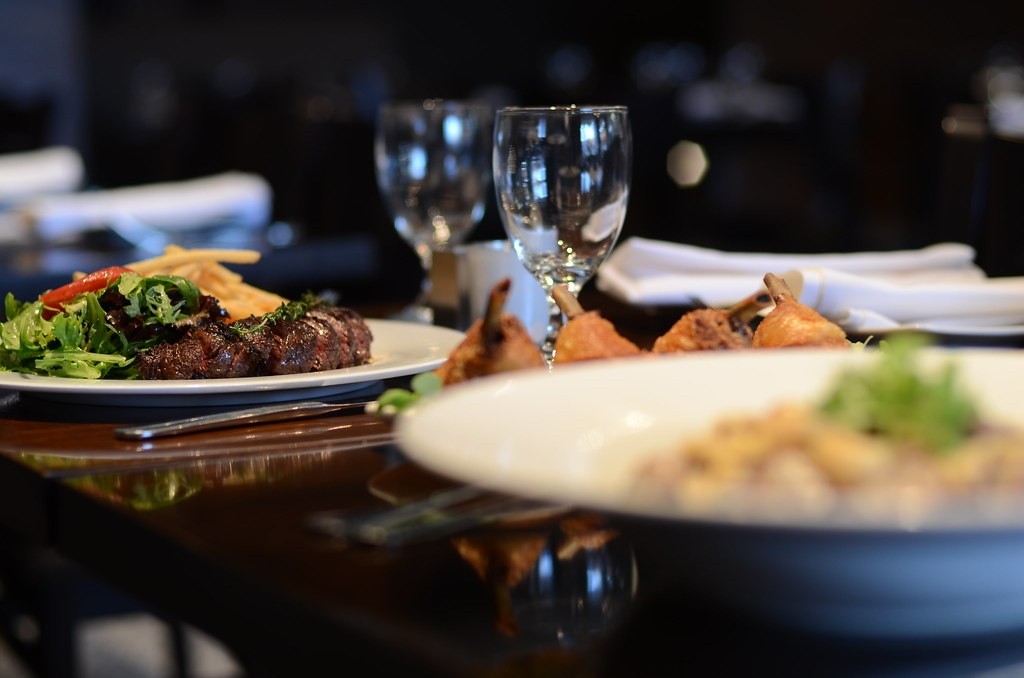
[0,274,204,380]
[822,327,985,458]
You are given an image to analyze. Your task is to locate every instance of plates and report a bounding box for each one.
[393,349,1022,635]
[1,320,471,407]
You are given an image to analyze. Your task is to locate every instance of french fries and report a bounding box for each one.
[70,242,291,321]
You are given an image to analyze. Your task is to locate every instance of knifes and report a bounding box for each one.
[112,390,403,442]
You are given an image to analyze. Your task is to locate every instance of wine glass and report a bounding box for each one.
[487,104,631,371]
[374,98,497,320]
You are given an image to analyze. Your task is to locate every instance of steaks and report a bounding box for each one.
[139,300,376,381]
